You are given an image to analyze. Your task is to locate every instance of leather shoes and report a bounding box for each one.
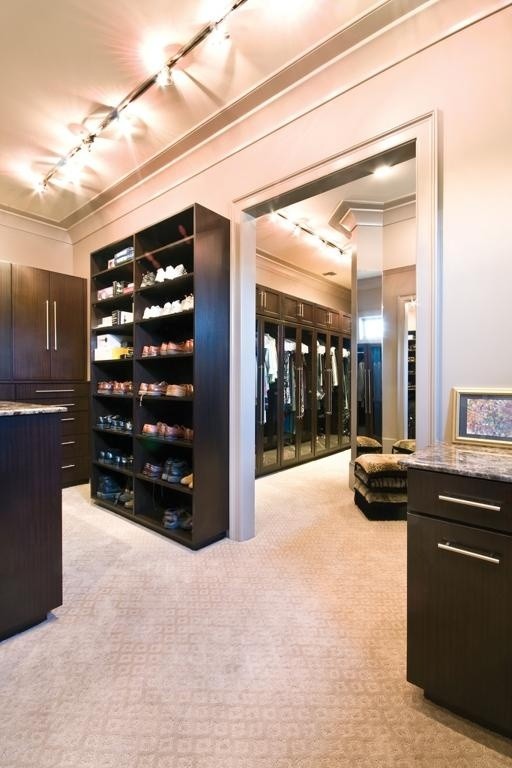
[93,263,193,534]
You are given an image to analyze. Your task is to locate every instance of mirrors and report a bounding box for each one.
[352,264,417,459]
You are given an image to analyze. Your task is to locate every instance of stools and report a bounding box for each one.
[392,438,417,454]
[353,453,407,522]
[358,436,382,456]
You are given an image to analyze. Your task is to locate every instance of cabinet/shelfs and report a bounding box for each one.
[89,202,230,555]
[358,343,381,434]
[254,281,359,476]
[1,258,88,490]
[2,398,70,645]
[396,438,511,741]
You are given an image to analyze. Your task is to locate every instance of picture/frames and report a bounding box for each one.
[450,387,512,450]
